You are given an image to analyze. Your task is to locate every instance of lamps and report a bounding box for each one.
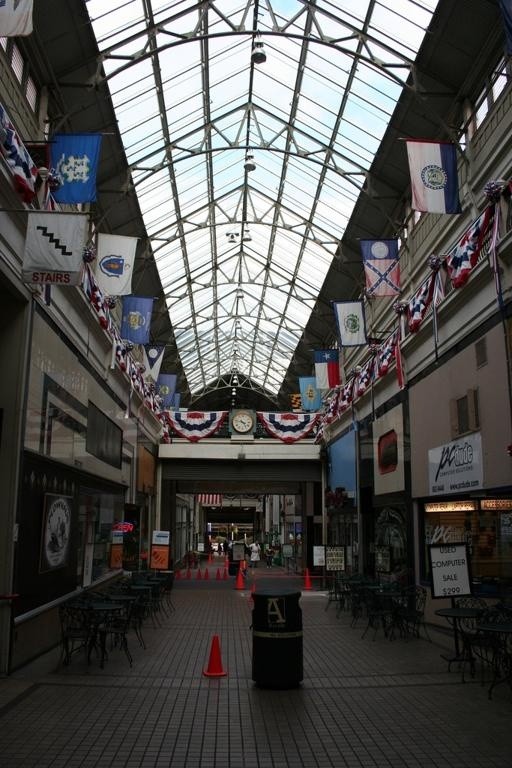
[244,148,256,170]
[242,222,252,241]
[250,29,266,63]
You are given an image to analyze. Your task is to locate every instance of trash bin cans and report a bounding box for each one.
[251,589,304,689]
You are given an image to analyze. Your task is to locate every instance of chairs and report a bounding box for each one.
[454,595,512,700]
[325,568,433,643]
[53,568,176,673]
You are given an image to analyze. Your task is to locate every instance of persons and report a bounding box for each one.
[243,539,281,569]
[208,538,235,556]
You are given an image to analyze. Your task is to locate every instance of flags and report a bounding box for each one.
[0,0,34,38]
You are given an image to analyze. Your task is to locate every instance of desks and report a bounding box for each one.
[435,608,484,679]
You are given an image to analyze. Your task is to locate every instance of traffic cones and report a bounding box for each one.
[303,568,314,590]
[175,552,229,581]
[203,634,227,677]
[233,568,246,590]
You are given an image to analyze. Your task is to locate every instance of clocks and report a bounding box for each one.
[231,412,254,433]
